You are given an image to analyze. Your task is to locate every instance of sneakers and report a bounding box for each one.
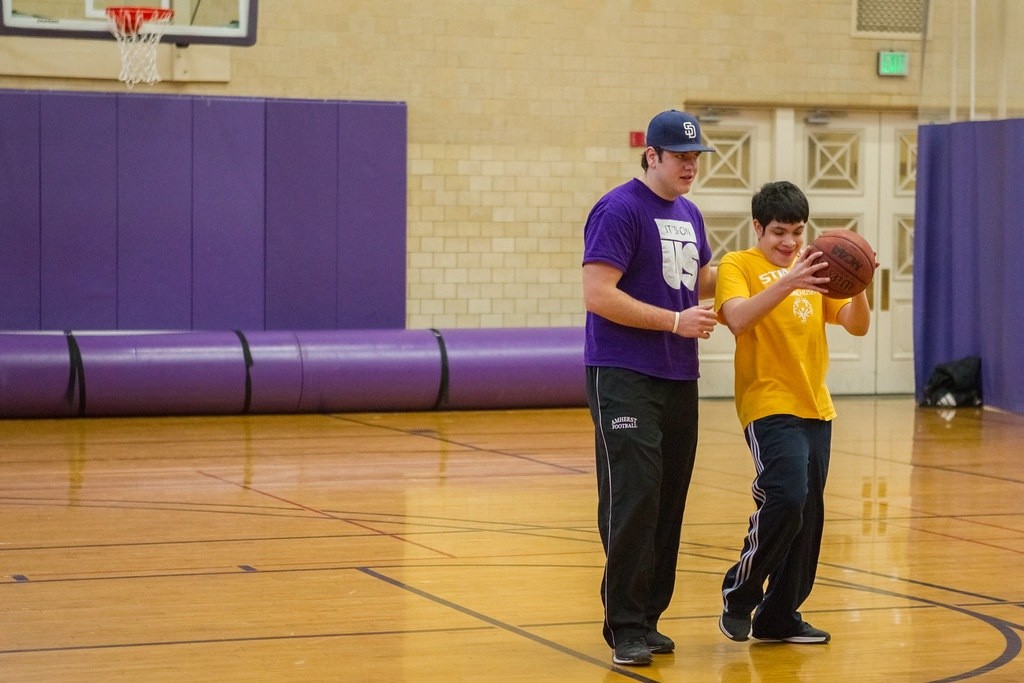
[645,631,675,653]
[751,621,831,643]
[719,605,758,642]
[612,638,653,664]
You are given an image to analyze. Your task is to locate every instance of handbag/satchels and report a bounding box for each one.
[919,357,983,408]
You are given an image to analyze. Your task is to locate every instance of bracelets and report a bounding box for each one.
[672,311,680,333]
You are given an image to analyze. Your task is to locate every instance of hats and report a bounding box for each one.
[646,110,715,152]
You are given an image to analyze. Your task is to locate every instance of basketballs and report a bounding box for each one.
[804,224,879,303]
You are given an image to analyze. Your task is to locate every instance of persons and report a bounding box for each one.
[714,181,880,643]
[582,110,803,664]
